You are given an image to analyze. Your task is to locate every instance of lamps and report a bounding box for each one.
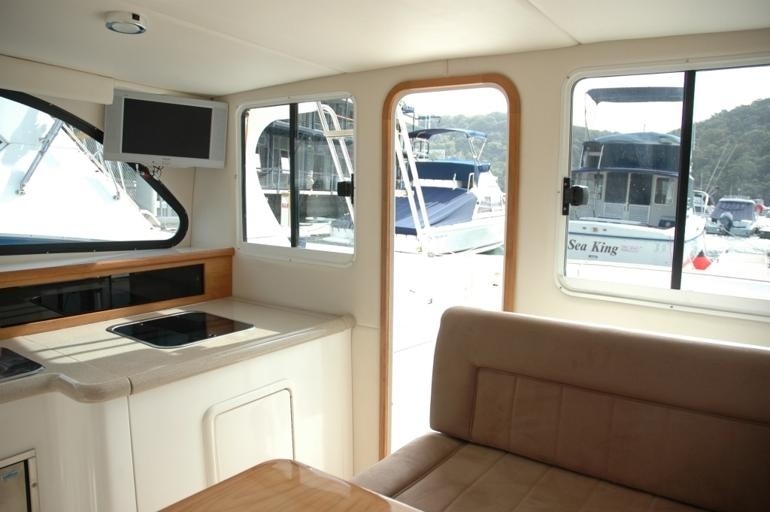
[105,11,146,34]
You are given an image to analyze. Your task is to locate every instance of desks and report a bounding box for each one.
[154,457,420,512]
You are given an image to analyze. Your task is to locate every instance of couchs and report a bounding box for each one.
[345,304,770,512]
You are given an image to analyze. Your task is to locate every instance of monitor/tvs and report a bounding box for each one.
[102,89,229,171]
